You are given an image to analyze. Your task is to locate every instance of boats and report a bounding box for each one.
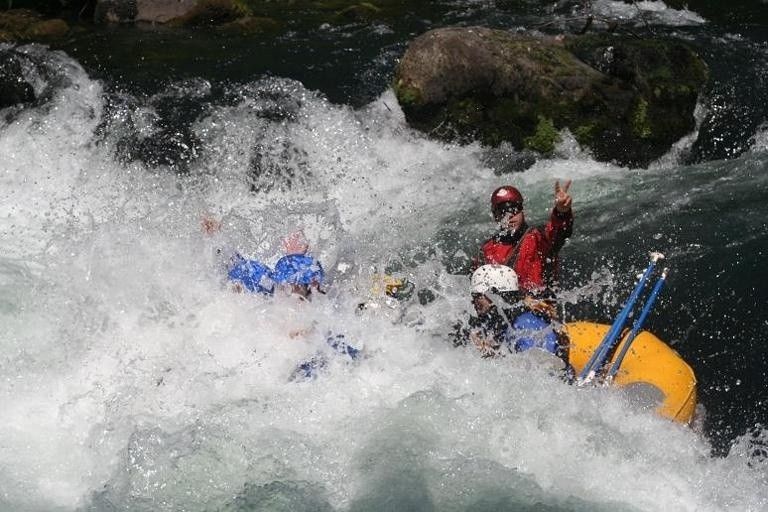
[555,321,698,428]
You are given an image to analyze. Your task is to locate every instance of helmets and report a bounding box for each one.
[226,257,278,298]
[469,260,521,294]
[274,252,326,287]
[490,184,527,208]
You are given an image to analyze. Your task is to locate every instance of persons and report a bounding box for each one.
[466,177,575,300]
[280,232,312,256]
[201,217,326,305]
[462,261,576,388]
